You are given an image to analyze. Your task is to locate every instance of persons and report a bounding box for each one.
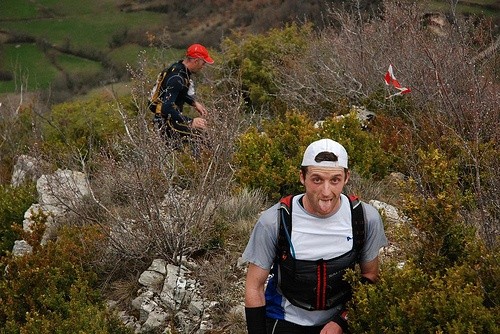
[148,43,214,149]
[241,138,388,334]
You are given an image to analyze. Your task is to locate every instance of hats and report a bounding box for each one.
[185,44,215,65]
[301,138,348,169]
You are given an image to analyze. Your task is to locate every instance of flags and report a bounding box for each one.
[385,64,411,95]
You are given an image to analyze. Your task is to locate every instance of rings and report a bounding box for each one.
[203,108,205,111]
